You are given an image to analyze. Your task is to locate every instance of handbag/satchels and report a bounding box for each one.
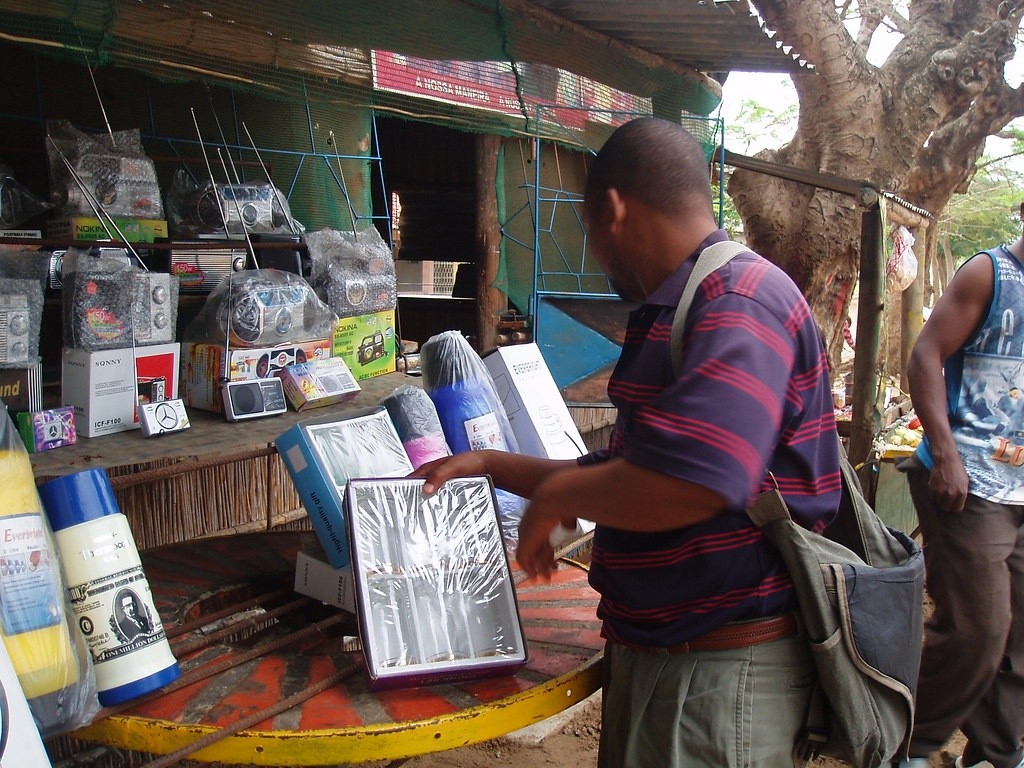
[669,241,925,768]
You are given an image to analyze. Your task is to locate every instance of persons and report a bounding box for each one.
[895,201,1024,768]
[404,117,842,768]
[841,316,855,362]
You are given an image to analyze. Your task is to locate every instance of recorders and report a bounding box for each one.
[0,15,396,439]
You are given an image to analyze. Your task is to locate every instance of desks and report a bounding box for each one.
[870,449,921,539]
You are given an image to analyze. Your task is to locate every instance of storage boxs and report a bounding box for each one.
[61,342,181,439]
[46,216,168,242]
[176,340,332,413]
[17,408,78,450]
[272,406,417,575]
[277,357,361,413]
[328,309,399,383]
[292,551,358,614]
[482,341,596,546]
[341,473,530,690]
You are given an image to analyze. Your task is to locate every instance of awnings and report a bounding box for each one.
[546,0,817,73]
